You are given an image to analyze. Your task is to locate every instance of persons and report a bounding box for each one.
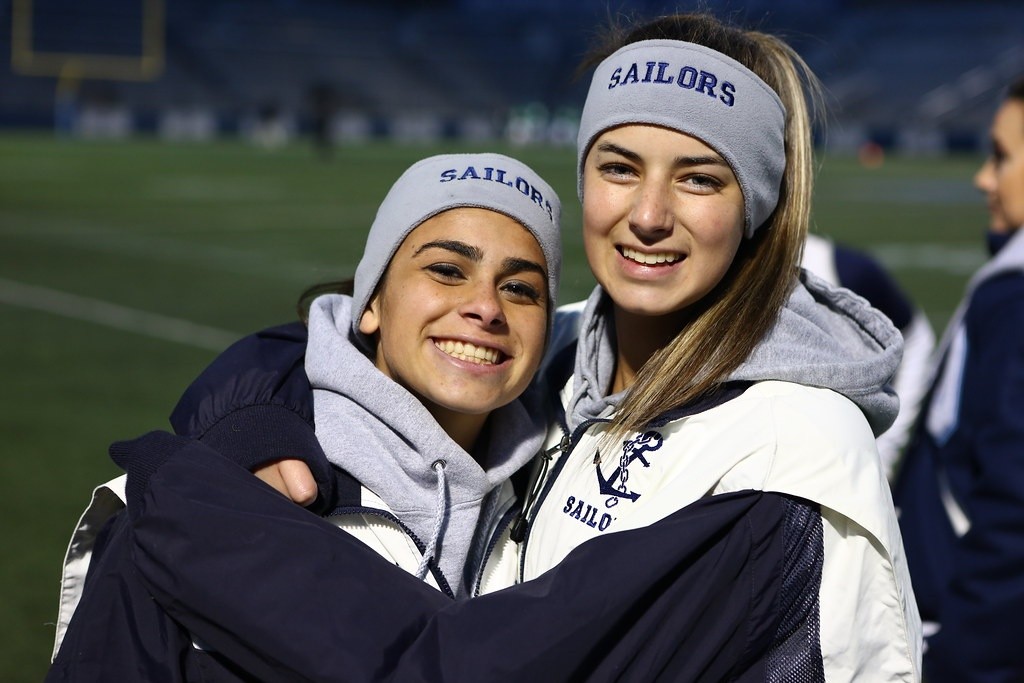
[891,72,1024,683]
[799,232,935,489]
[105,13,924,683]
[48,152,785,683]
